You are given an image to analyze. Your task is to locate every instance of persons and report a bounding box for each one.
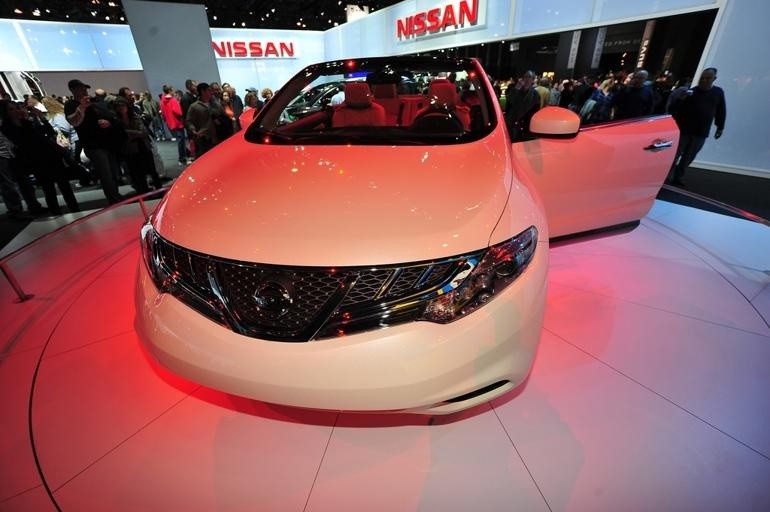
[273,105,296,127]
[1,77,281,222]
[418,68,726,181]
[330,83,349,108]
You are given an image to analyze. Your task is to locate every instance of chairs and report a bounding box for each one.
[331,79,473,135]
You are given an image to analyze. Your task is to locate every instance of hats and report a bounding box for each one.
[246,87,258,94]
[68,79,92,89]
[104,95,130,108]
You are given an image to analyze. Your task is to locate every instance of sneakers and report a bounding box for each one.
[179,157,195,167]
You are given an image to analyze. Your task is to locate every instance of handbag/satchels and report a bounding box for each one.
[56,132,72,151]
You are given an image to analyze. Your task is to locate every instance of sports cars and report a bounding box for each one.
[137,47,683,420]
